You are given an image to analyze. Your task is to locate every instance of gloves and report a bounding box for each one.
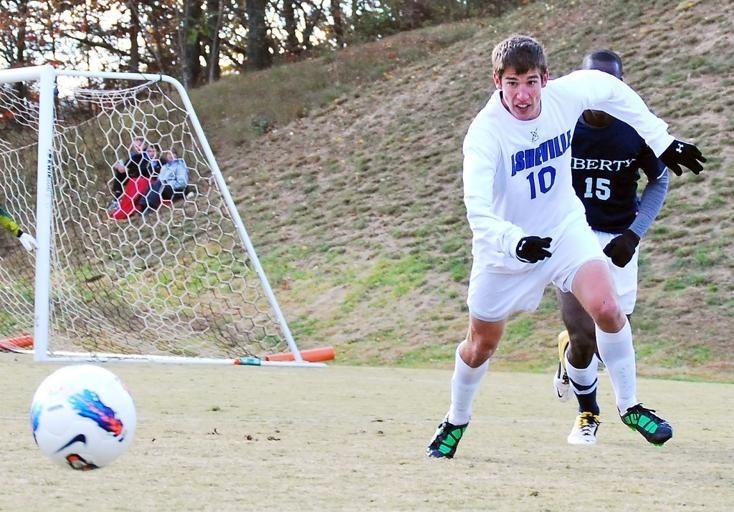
[16,229,39,252]
[516,235,552,263]
[603,229,640,268]
[660,139,707,176]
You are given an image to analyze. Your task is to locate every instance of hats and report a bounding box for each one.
[583,48,623,76]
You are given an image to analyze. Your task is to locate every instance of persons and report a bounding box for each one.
[111,143,167,219]
[105,137,151,214]
[0,205,37,253]
[548,46,673,449]
[423,33,707,463]
[132,147,190,218]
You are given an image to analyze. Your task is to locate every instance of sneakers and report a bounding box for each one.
[619,403,672,447]
[427,412,470,460]
[552,329,573,403]
[568,411,600,447]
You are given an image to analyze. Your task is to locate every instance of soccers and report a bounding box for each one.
[31,363,135,471]
